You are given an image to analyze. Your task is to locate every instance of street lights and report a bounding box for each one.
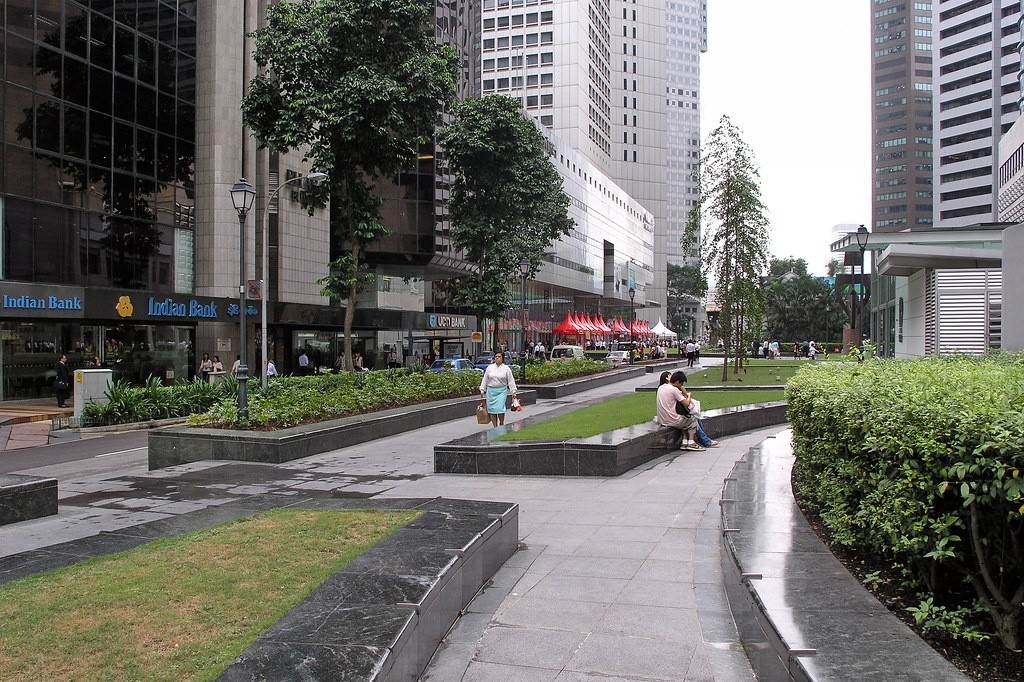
[856,224,869,349]
[663,323,666,340]
[826,304,829,344]
[629,288,635,364]
[678,304,681,359]
[550,312,555,343]
[229,177,256,418]
[518,257,531,383]
[260,173,330,397]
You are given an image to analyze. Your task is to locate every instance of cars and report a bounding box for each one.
[422,359,483,375]
[473,355,522,371]
[605,352,634,364]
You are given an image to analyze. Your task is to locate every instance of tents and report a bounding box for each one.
[553,310,677,350]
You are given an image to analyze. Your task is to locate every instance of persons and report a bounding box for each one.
[231,355,241,379]
[860,334,871,360]
[656,370,706,451]
[479,350,518,428]
[93,357,122,369]
[660,372,719,448]
[56,355,73,408]
[336,339,821,372]
[267,359,278,383]
[212,356,223,372]
[198,353,212,383]
[299,349,309,376]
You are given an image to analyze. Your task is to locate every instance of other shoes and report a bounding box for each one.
[60,404,69,408]
[711,440,719,446]
[680,443,707,451]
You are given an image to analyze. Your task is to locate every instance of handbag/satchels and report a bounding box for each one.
[476,393,490,425]
[676,401,692,418]
[510,395,523,412]
[686,398,701,415]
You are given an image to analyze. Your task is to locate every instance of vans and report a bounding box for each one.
[550,345,586,362]
[618,342,639,360]
